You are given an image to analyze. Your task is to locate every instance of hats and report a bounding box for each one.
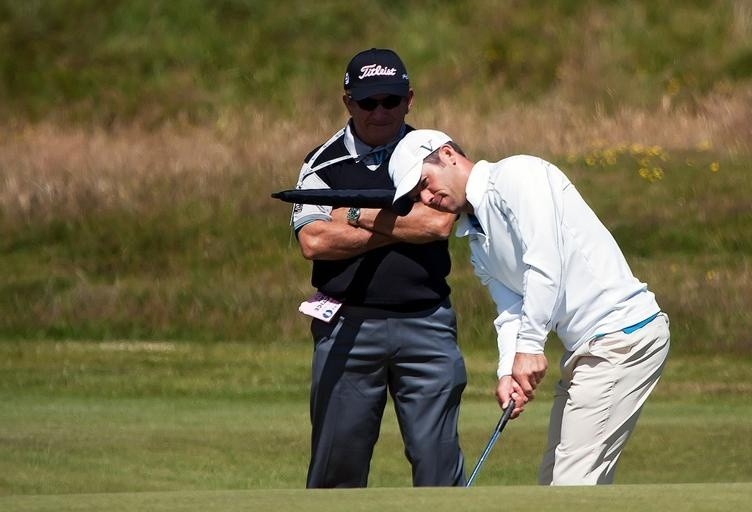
[388,128,453,217]
[343,46,410,101]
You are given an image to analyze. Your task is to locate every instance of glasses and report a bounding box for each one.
[356,99,405,110]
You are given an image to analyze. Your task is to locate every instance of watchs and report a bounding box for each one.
[346,207,361,227]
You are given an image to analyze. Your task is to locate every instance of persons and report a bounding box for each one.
[288,48,467,489]
[386,129,671,486]
